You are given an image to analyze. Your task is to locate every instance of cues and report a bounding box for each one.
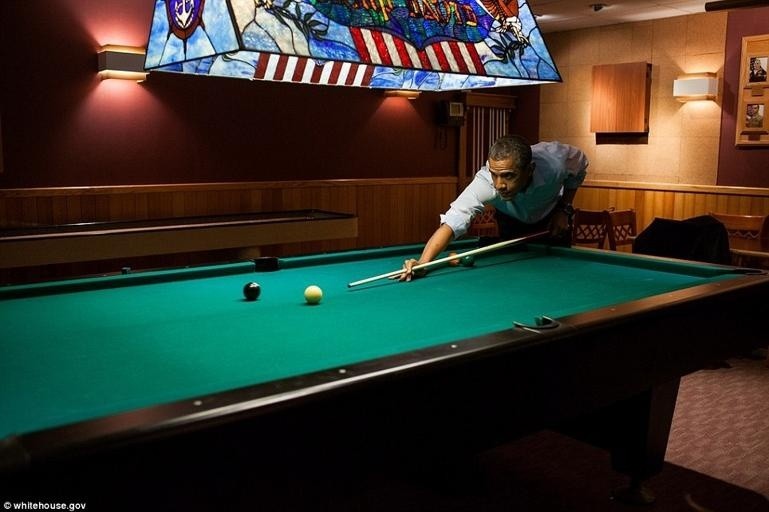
[348,230,549,287]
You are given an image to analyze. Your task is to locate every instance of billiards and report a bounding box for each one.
[243,282,260,300]
[448,252,460,267]
[304,285,322,303]
[462,250,475,267]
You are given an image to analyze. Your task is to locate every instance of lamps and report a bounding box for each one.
[382,87,422,101]
[95,43,152,84]
[672,72,719,101]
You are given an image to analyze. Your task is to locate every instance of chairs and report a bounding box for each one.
[602,208,638,251]
[469,204,499,236]
[708,211,766,251]
[572,206,615,249]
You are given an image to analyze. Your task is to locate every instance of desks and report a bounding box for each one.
[0,238,769,512]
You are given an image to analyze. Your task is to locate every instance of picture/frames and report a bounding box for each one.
[734,33,769,147]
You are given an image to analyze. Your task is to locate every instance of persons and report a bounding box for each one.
[388,136,588,282]
[751,60,767,83]
[747,105,762,127]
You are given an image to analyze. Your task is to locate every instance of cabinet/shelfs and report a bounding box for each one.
[589,61,652,133]
